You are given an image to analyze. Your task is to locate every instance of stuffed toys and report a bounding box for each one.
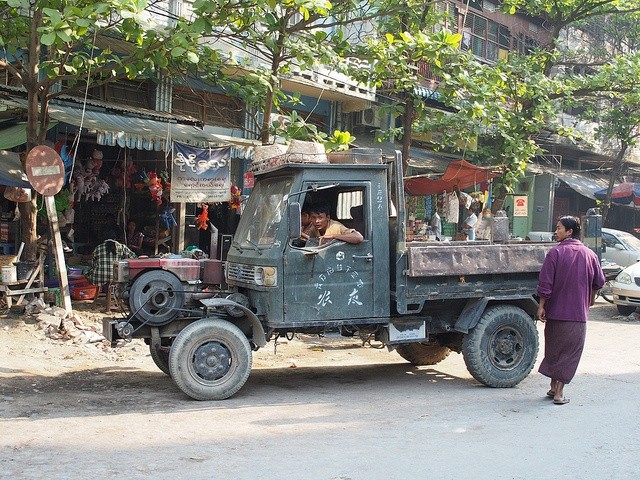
[135,165,181,205]
[197,204,209,231]
[54,139,135,203]
[158,204,178,230]
[36,188,75,227]
[227,185,243,210]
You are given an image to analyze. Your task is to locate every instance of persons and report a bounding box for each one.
[537,215,606,405]
[308,203,364,245]
[85,229,138,287]
[96,213,123,246]
[460,206,478,241]
[300,206,314,241]
[430,208,441,241]
[120,220,144,256]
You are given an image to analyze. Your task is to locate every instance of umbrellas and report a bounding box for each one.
[594,181,640,236]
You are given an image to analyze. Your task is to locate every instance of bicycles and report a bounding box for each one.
[594,259,623,303]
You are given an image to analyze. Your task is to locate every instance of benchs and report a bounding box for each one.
[89,281,117,312]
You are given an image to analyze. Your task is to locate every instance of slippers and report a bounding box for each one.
[547,390,555,395]
[554,396,570,404]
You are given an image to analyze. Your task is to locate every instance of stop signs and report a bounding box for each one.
[25,145,65,196]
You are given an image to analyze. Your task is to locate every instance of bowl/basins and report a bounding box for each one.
[70,286,102,300]
[45,266,88,287]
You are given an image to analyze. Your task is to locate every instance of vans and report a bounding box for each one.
[601,228,640,269]
[613,262,640,316]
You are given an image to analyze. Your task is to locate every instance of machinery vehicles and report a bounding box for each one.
[104,149,560,401]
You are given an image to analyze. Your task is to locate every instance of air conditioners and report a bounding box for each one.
[351,104,382,128]
